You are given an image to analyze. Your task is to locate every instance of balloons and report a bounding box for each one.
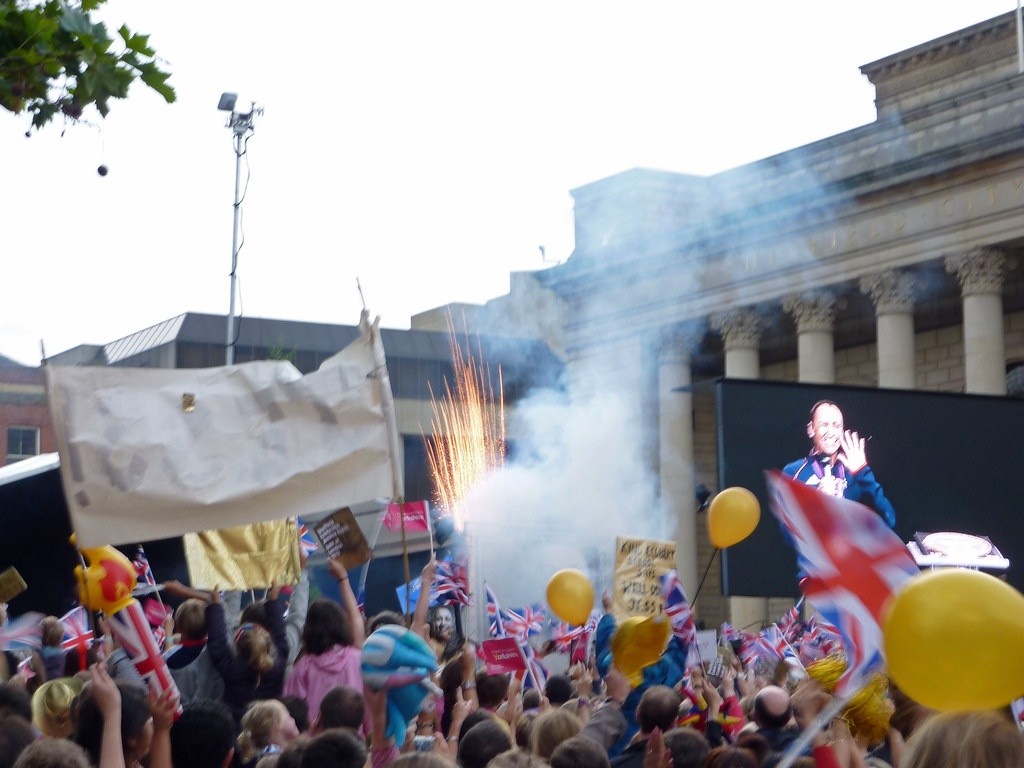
[706,485,761,550]
[547,568,595,628]
[884,566,1024,712]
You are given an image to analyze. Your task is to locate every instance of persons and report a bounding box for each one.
[775,399,897,584]
[1,545,1024,768]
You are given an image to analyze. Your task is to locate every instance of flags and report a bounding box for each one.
[660,468,924,700]
[0,499,602,695]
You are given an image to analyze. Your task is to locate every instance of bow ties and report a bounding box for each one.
[819,459,831,469]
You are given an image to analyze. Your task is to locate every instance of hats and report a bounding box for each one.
[31,676,85,739]
